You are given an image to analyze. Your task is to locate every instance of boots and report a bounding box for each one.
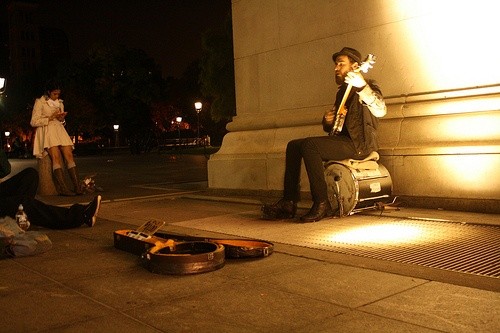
[54,167,76,196]
[69,166,86,195]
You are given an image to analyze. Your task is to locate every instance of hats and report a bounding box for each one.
[332,47,362,67]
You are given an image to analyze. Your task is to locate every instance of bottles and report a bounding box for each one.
[16,205,27,231]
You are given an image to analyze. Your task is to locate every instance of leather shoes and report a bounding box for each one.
[299,200,333,222]
[261,198,298,219]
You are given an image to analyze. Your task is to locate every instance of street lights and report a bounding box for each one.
[5,131,10,147]
[176,116,182,150]
[194,101,203,138]
[113,124,119,147]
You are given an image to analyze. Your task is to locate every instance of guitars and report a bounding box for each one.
[329,51,376,135]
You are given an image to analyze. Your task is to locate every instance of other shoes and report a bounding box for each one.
[83,194,102,228]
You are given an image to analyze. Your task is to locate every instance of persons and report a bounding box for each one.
[0,156,101,228]
[30,83,86,195]
[262,46,387,222]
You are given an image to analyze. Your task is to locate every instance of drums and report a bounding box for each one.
[324,161,394,218]
